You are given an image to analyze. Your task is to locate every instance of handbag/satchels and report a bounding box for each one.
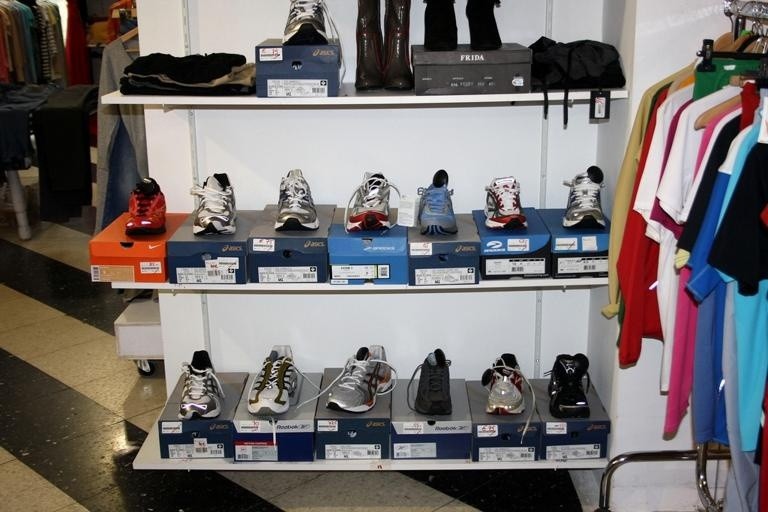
[529,36,627,131]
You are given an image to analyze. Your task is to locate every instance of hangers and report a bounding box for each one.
[120,27,139,52]
[694,8,768,134]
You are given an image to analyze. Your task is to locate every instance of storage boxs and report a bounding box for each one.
[408,213,481,286]
[405,45,536,93]
[327,208,408,286]
[472,208,551,279]
[464,379,545,465]
[248,205,336,282]
[86,212,190,284]
[234,372,324,462]
[316,367,391,460]
[391,379,472,462]
[529,376,611,462]
[168,209,266,285]
[255,39,340,97]
[534,209,618,278]
[156,368,250,464]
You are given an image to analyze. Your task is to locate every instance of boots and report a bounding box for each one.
[465,0,504,53]
[354,0,414,91]
[421,0,459,52]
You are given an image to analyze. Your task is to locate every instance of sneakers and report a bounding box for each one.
[545,351,590,420]
[324,343,393,414]
[245,344,298,414]
[176,346,222,421]
[415,168,459,236]
[485,351,527,416]
[188,171,238,235]
[272,166,321,231]
[345,171,398,232]
[121,175,168,236]
[278,0,330,46]
[412,348,453,416]
[560,165,608,231]
[482,174,531,231]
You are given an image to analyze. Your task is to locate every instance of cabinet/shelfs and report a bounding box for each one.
[102,76,625,474]
